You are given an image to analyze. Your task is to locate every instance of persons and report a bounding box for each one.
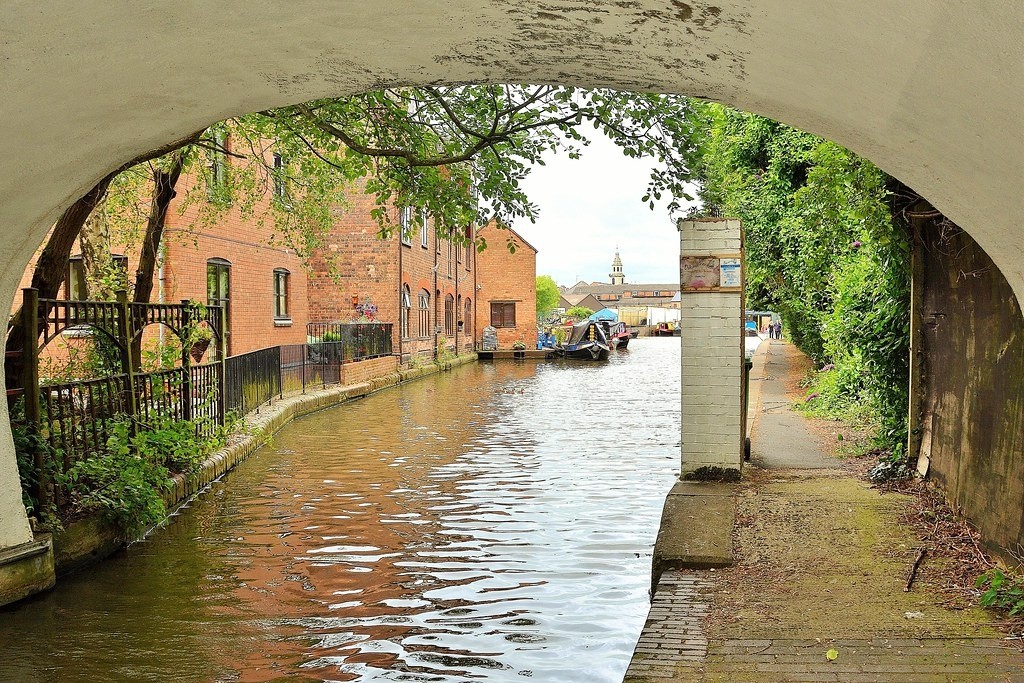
[761,319,782,340]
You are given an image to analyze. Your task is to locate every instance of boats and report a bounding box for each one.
[561,317,610,361]
[626,329,640,338]
[654,320,681,336]
[745,320,758,336]
[588,308,630,348]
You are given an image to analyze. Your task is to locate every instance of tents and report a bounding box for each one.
[589,308,618,323]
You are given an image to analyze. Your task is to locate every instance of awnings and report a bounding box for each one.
[620,307,648,312]
